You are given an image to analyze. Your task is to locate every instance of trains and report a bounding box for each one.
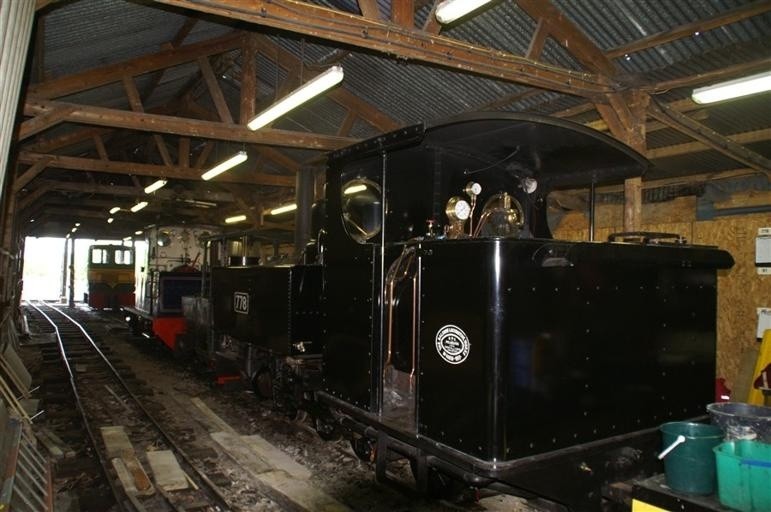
[74,102,737,502]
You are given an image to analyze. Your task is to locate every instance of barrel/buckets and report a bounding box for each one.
[712,439,771,512]
[659,421,724,496]
[707,401,771,441]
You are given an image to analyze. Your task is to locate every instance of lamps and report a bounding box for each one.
[690,70,771,106]
[247,66,345,134]
[435,0,495,26]
[201,145,249,184]
